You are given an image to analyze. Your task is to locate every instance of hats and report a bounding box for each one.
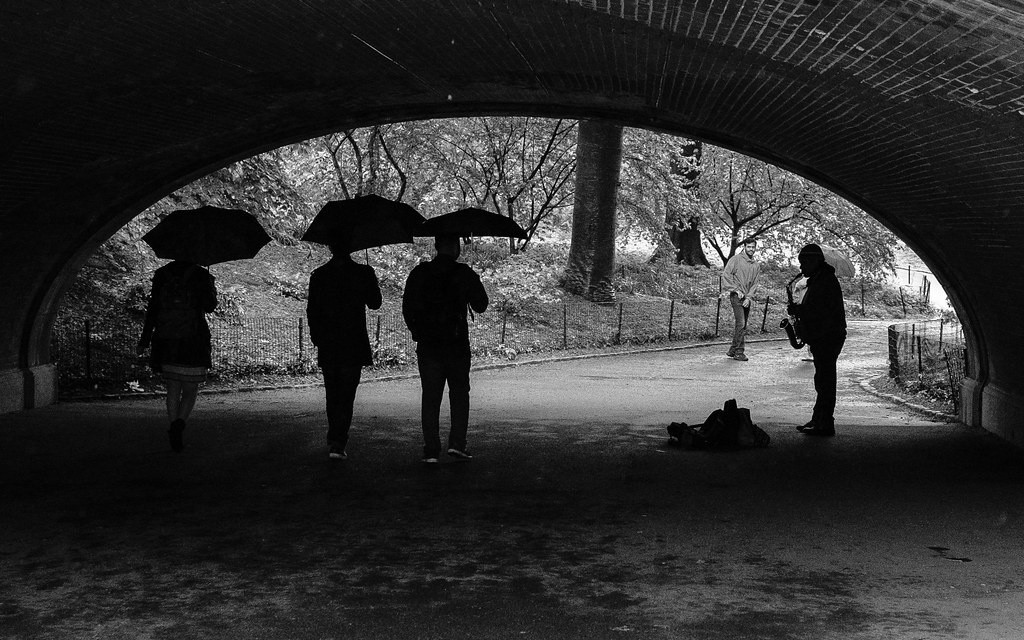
[800,244,824,257]
[435,226,460,244]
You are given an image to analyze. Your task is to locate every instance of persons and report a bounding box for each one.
[793,277,814,361]
[306,254,382,460]
[136,262,216,453]
[722,238,761,361]
[402,236,489,463]
[786,244,847,435]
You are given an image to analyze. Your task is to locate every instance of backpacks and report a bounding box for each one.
[154,266,205,340]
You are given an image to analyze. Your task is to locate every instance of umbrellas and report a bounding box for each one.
[413,207,530,270]
[819,244,855,277]
[141,205,274,273]
[300,193,428,253]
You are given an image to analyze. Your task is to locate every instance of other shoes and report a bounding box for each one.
[797,421,816,432]
[168,417,185,453]
[803,426,835,437]
[734,353,748,361]
[419,452,439,463]
[328,443,348,460]
[449,447,472,459]
[726,347,735,357]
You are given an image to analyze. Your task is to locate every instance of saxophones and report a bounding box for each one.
[778,271,808,350]
